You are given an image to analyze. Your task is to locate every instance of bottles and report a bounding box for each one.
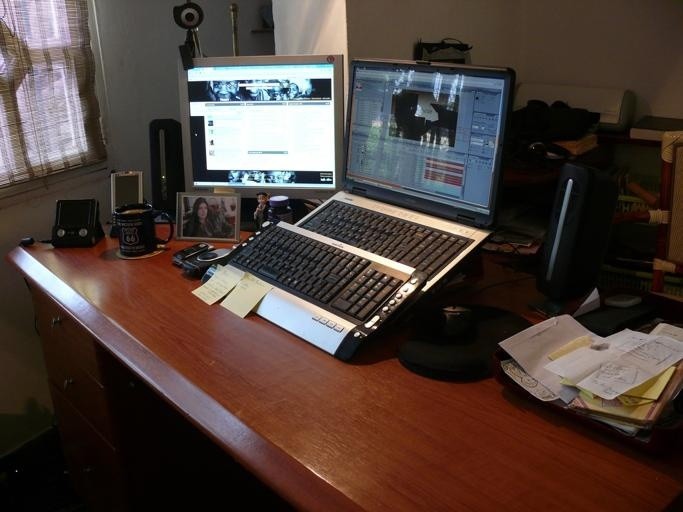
[267,195,292,225]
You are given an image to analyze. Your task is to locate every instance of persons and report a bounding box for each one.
[253,192,267,230]
[228,170,296,183]
[182,197,217,237]
[207,196,235,238]
[209,80,298,101]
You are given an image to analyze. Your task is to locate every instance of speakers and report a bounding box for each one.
[149,119,185,224]
[536,163,612,301]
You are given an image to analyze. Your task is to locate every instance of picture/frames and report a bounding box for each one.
[176,191,241,244]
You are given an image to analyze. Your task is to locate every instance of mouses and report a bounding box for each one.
[438,303,478,346]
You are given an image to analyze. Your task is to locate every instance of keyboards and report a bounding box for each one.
[209,219,427,363]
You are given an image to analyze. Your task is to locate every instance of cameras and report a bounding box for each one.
[111,169,143,228]
[52,199,99,248]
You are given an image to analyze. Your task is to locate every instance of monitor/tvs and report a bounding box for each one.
[178,54,345,200]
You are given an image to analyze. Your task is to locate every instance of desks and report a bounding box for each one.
[5,216,683,512]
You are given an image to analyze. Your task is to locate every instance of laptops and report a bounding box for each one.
[293,59,517,326]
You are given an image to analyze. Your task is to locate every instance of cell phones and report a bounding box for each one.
[183,248,234,279]
[172,241,215,267]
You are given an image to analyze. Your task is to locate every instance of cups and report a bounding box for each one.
[111,202,173,256]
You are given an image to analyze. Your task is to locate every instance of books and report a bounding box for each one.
[479,208,548,255]
[542,316,683,445]
